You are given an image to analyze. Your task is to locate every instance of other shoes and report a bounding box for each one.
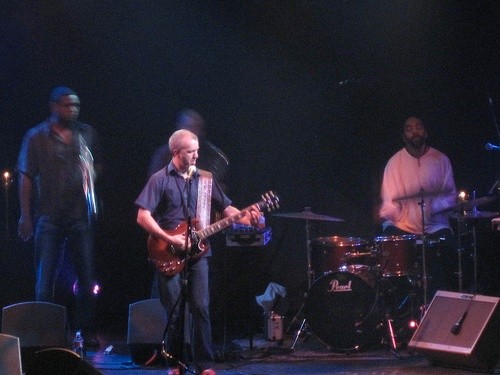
[168,364,185,375]
[196,368,216,375]
[81,333,100,348]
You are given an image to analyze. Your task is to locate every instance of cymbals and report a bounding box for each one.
[451,212,499,223]
[267,209,347,223]
[434,195,496,214]
[392,188,453,202]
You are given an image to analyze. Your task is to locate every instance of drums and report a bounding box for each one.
[310,236,371,283]
[374,235,416,277]
[303,264,400,351]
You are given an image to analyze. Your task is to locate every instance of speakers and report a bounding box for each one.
[126,299,169,362]
[408,290,500,373]
[0,300,104,375]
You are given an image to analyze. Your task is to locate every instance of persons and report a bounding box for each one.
[16,86,101,352]
[376,113,456,261]
[134,110,260,375]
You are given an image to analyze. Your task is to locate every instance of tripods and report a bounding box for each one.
[285,219,317,351]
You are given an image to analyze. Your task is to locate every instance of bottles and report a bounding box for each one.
[72,331,84,357]
[266,296,285,343]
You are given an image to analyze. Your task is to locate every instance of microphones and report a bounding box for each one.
[450,311,467,335]
[485,142,500,151]
[187,165,197,180]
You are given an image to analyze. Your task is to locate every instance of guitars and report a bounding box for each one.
[147,190,281,278]
